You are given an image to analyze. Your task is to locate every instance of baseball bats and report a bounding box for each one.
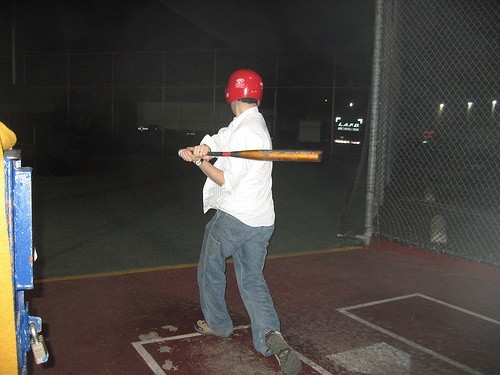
[206,150,323,162]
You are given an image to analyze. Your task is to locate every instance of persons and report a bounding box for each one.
[177,68,302,375]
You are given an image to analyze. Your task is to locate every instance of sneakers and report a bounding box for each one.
[194,320,233,337]
[265,330,302,375]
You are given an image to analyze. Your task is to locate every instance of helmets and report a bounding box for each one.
[225,70,263,104]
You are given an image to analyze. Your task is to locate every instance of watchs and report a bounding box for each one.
[196,158,204,166]
